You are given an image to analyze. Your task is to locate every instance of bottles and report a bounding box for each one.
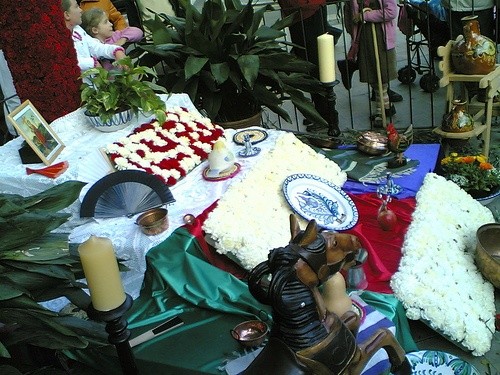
[445,99,474,132]
[449,14,498,76]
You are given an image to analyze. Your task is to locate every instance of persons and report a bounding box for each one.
[27,120,53,150]
[80,9,143,79]
[442,0,495,105]
[62,0,129,91]
[79,0,127,30]
[278,0,339,132]
[337,60,404,101]
[344,0,397,126]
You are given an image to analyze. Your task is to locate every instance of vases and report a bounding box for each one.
[442,98,474,132]
[472,185,500,205]
[451,16,497,75]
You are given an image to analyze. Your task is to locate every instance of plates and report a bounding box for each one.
[283,173,358,231]
[389,350,481,375]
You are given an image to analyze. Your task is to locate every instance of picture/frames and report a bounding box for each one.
[7,99,65,166]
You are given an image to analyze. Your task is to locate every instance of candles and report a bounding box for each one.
[78,235,126,312]
[213,141,224,153]
[317,32,336,83]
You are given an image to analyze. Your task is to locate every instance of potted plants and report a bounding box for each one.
[78,56,168,132]
[124,0,329,130]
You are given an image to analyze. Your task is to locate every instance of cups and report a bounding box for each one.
[231,311,269,348]
[135,207,168,234]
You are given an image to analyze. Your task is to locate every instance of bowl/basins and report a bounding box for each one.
[475,224,500,287]
[474,189,500,205]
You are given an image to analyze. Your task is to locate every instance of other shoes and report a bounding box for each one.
[337,59,352,90]
[372,88,403,102]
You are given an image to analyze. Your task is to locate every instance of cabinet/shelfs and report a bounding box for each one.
[431,35,500,162]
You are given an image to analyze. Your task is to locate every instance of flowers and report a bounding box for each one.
[202,131,348,273]
[389,171,495,357]
[0,0,84,124]
[104,105,223,187]
[436,153,500,191]
